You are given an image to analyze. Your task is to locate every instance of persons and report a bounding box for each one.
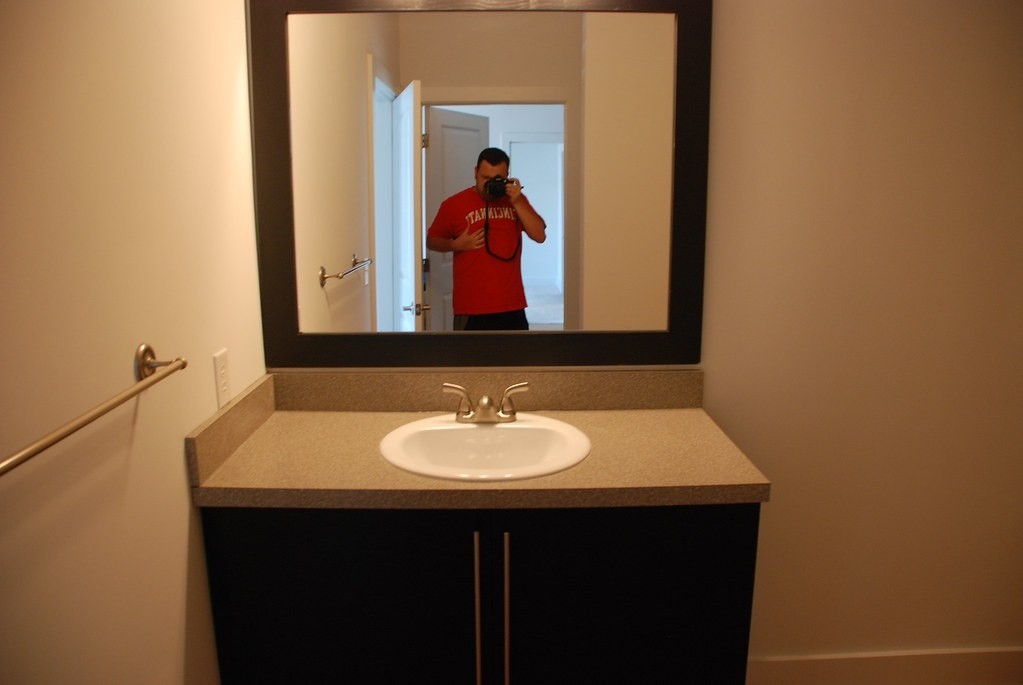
[425,147,546,331]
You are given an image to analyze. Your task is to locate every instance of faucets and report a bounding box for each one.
[476,394,497,422]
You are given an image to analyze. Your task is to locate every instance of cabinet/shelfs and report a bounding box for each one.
[201,503,763,685]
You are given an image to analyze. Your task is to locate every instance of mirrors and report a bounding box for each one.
[246,0,715,365]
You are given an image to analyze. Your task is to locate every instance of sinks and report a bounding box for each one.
[379,413,592,481]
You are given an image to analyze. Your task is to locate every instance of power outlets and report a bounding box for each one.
[213,348,231,408]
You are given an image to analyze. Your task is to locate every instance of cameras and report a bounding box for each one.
[488,176,513,198]
